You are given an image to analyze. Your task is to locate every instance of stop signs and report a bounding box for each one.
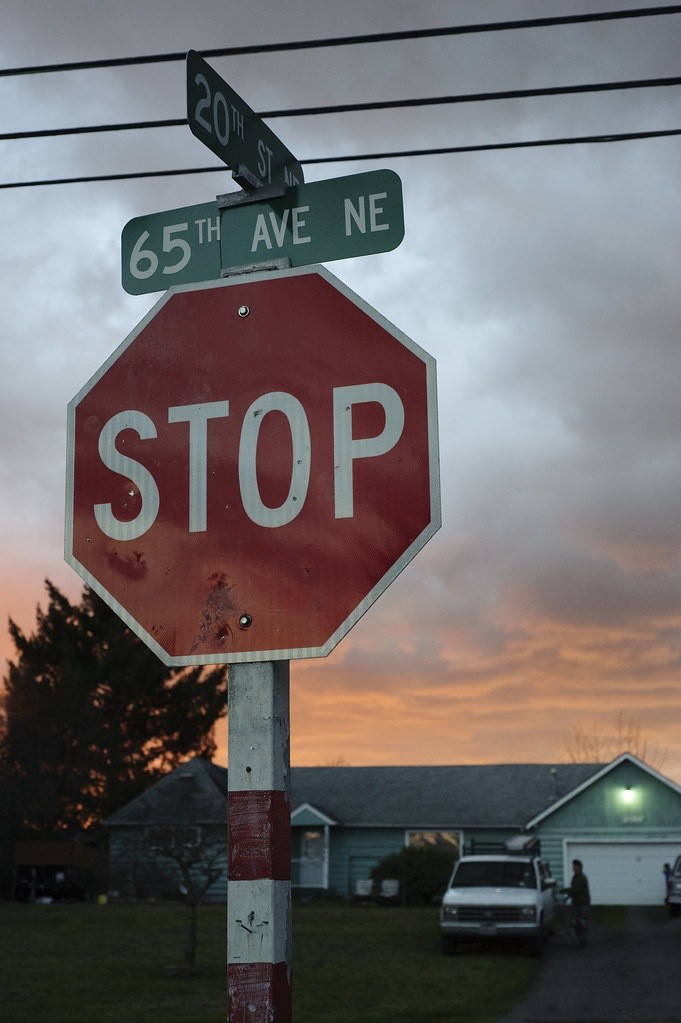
[62,265,445,668]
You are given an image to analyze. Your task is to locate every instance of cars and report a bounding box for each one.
[15,862,97,902]
[665,855,681,912]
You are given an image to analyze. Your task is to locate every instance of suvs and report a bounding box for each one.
[436,854,557,959]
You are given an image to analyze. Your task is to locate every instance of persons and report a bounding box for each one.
[557,860,591,950]
[663,863,672,903]
